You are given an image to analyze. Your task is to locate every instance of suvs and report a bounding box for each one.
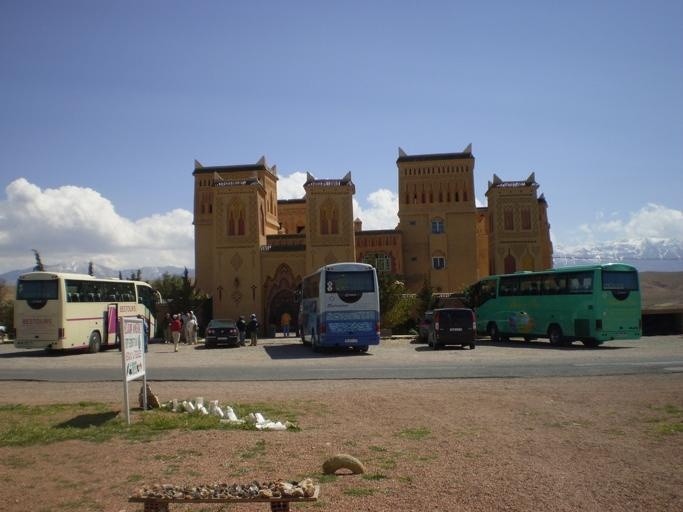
[427,308,477,350]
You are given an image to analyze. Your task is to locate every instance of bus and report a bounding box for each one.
[12,271,163,355]
[464,262,643,348]
[291,261,380,354]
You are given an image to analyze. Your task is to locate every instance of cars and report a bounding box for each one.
[415,311,433,343]
[204,319,240,349]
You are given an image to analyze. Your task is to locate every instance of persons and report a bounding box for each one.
[163,310,199,352]
[237,316,247,346]
[137,315,148,352]
[247,313,259,346]
[281,311,291,337]
